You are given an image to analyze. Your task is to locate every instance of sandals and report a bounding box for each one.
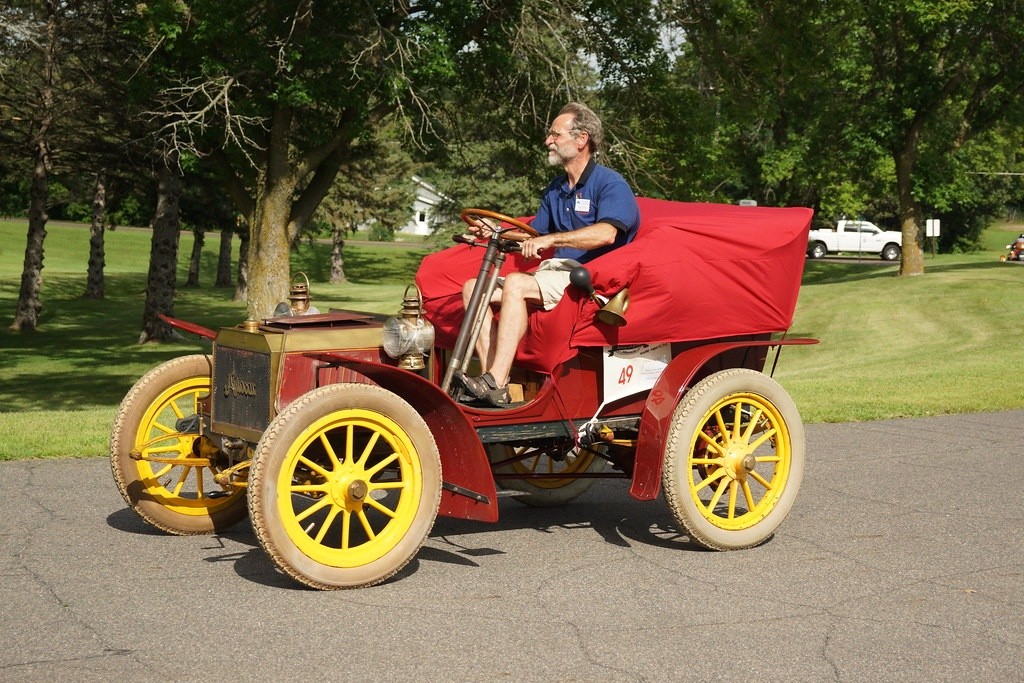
[453,370,512,408]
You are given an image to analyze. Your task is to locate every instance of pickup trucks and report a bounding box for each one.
[807,219,902,261]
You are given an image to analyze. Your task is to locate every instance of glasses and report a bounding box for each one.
[544,128,581,136]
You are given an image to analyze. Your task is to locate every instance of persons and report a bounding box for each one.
[1010,233,1024,245]
[453,103,641,406]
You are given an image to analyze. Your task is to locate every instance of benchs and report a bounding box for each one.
[415,195,815,394]
